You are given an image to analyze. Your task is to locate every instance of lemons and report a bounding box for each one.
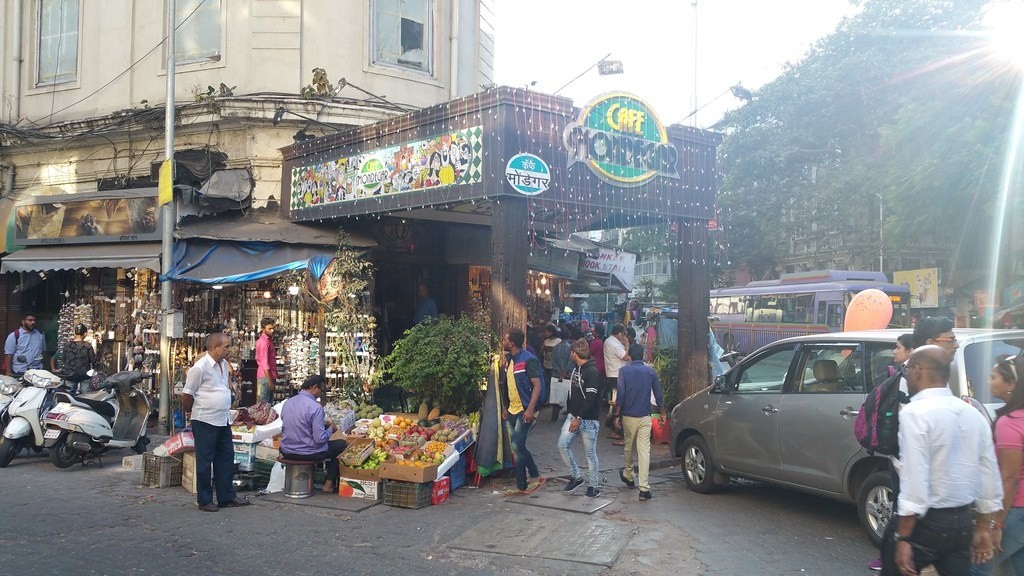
[348,460,380,469]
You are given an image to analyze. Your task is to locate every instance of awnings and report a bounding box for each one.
[0,242,162,274]
[159,239,373,285]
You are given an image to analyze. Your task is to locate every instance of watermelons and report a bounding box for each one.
[417,419,439,426]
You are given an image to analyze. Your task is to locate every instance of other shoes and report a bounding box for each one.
[608,433,624,439]
[868,559,883,571]
[218,496,250,507]
[611,441,625,446]
[584,486,601,499]
[619,467,635,487]
[563,477,585,494]
[639,491,651,501]
[198,502,218,512]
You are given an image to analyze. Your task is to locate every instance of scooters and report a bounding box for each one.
[0,354,75,469]
[39,368,154,469]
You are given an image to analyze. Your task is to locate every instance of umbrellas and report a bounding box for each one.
[466,355,516,489]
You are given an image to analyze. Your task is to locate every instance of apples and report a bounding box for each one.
[403,425,435,440]
[374,440,412,452]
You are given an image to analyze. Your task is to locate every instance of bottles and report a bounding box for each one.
[231,378,239,407]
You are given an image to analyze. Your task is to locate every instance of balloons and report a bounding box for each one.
[841,288,893,359]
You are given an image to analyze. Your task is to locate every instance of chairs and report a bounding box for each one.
[804,360,843,393]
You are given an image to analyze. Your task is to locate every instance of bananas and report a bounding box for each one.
[370,449,389,462]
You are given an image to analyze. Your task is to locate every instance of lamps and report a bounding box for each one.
[679,81,753,124]
[271,107,342,132]
[553,51,634,99]
[329,78,407,112]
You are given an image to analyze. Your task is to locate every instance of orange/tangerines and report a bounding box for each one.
[393,416,417,430]
[392,460,427,467]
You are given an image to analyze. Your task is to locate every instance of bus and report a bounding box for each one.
[710,269,912,363]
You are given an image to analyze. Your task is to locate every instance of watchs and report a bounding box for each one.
[613,412,619,418]
[892,531,911,542]
[988,519,1005,529]
[576,415,582,420]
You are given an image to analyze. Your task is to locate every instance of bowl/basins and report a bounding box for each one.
[232,480,247,491]
[313,468,338,489]
[233,459,241,472]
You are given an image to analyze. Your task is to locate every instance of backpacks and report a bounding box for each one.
[1,328,43,372]
[854,362,910,463]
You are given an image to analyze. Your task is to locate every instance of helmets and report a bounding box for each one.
[91,375,105,391]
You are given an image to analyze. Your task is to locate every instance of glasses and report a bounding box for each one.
[24,319,36,321]
[933,337,957,345]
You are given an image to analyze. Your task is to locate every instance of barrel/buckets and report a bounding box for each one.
[285,464,314,499]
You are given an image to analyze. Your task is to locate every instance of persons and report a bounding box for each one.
[868,316,961,574]
[50,323,101,396]
[411,280,438,328]
[969,353,1024,576]
[525,304,658,445]
[881,344,1004,576]
[255,318,278,406]
[180,332,250,513]
[3,313,48,386]
[500,327,545,497]
[556,338,602,500]
[612,344,666,501]
[265,375,347,493]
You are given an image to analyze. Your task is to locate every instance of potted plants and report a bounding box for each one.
[648,336,678,445]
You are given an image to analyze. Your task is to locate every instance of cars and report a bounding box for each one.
[668,326,1023,552]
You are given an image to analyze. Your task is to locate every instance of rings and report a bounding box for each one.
[983,552,988,556]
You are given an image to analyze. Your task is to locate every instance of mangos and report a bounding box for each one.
[412,449,445,464]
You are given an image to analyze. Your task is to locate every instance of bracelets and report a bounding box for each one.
[971,520,989,529]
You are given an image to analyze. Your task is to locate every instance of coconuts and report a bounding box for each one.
[352,403,384,419]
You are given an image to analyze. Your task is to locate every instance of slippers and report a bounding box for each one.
[526,477,546,493]
[502,488,530,497]
[321,487,339,495]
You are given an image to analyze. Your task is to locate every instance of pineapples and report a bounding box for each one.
[431,428,458,442]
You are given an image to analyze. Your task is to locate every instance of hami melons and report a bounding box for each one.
[368,417,391,436]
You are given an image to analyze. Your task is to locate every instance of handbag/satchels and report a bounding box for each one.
[549,377,569,411]
[266,461,285,492]
[57,367,77,380]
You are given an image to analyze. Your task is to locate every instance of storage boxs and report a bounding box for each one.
[141,397,481,508]
